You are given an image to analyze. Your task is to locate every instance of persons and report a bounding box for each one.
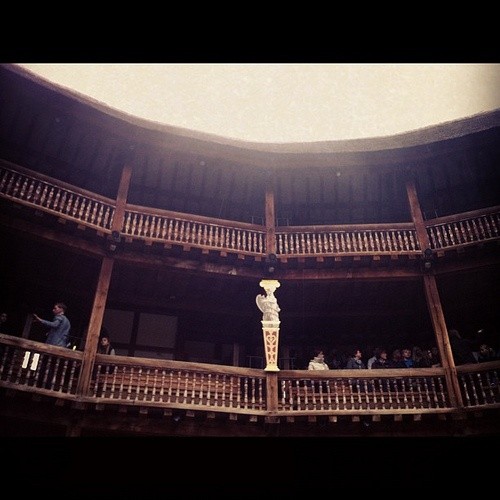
[30,302,71,389]
[308,329,500,413]
[1,312,9,380]
[93,336,116,398]
